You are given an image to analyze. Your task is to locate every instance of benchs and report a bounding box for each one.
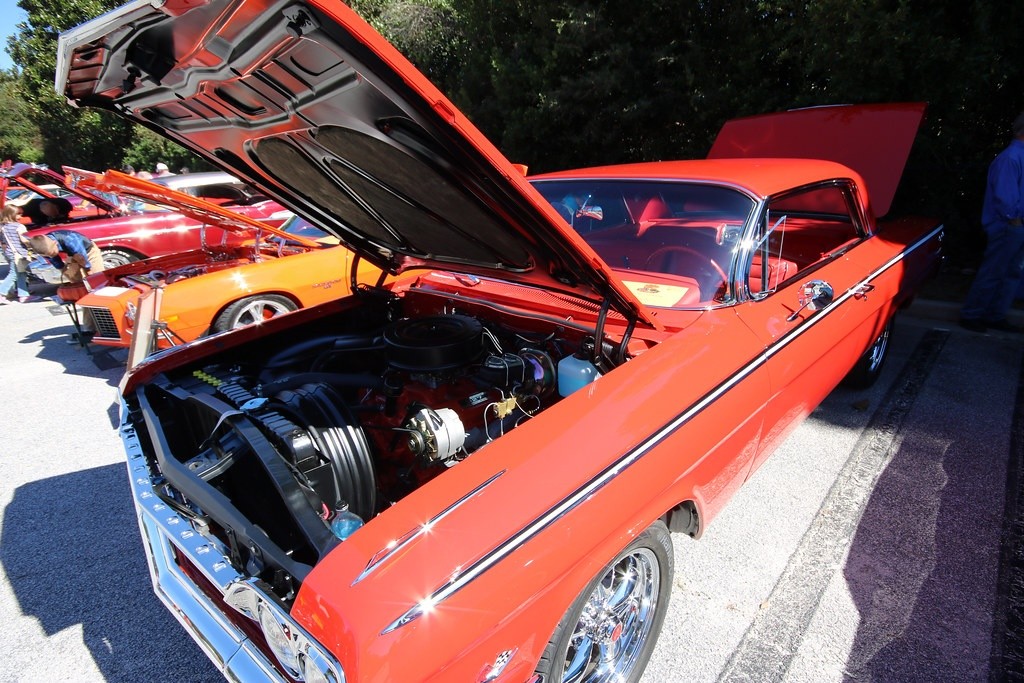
[585,222,798,294]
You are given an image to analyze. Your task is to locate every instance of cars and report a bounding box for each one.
[0,159,434,368]
[50,0,963,683]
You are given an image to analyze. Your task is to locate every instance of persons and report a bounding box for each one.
[959,111,1024,333]
[123,162,189,180]
[30,230,105,344]
[0,204,34,305]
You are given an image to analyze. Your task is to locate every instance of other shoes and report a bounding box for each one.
[0,295,10,305]
[18,295,41,302]
[958,318,987,332]
[982,316,1021,333]
[66,330,96,344]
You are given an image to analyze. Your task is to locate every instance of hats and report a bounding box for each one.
[155,163,168,173]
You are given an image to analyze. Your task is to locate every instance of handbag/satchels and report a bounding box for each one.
[13,252,33,273]
